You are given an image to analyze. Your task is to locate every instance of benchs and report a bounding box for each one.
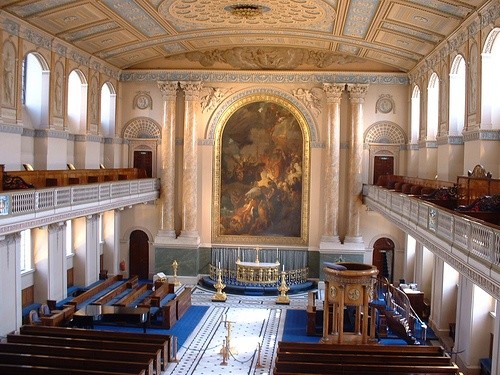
[273,342,464,375]
[0,325,173,375]
[22,274,191,329]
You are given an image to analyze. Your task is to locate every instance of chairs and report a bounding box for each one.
[388,180,433,198]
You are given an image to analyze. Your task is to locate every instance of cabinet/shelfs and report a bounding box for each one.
[401,287,424,320]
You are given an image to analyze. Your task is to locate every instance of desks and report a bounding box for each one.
[306,305,316,335]
